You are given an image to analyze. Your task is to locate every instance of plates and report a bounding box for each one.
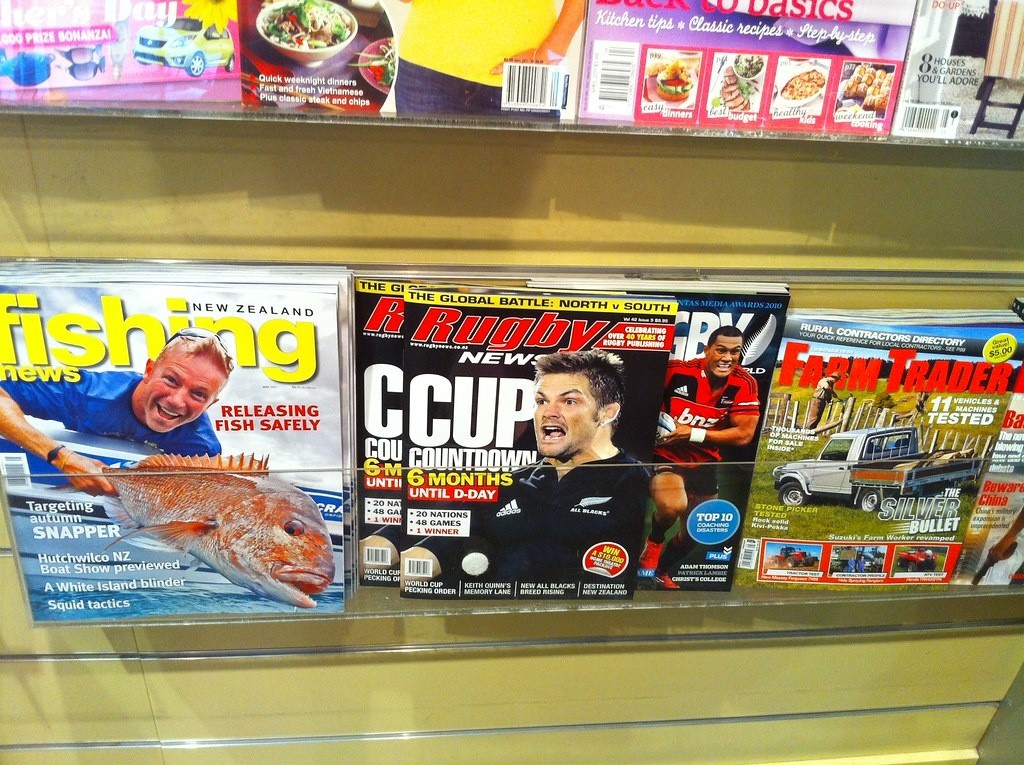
[358,38,390,95]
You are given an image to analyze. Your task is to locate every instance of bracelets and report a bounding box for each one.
[46,444,66,467]
[689,426,706,443]
[59,450,74,473]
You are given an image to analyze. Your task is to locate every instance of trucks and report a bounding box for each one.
[772,426,982,513]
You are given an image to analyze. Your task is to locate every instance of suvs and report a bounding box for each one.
[770,547,814,567]
[897,548,937,573]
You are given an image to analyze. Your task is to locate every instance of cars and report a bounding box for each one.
[133,16,234,77]
[831,550,880,571]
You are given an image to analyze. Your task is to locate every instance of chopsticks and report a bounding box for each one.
[241,72,345,112]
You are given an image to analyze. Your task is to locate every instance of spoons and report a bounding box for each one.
[239,43,294,86]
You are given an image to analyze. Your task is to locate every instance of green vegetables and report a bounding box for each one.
[348,38,396,87]
[264,0,347,50]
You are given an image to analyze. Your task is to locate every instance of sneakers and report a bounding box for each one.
[639,536,665,571]
[652,569,681,589]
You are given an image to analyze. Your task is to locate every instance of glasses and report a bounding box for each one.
[57,42,103,65]
[163,326,233,372]
[55,58,106,81]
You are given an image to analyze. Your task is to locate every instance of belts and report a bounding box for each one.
[812,396,825,401]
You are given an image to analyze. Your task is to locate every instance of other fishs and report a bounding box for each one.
[49,454,335,610]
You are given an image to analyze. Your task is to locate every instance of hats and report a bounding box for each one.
[832,372,842,380]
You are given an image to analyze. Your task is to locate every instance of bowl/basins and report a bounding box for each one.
[733,54,765,81]
[775,65,829,108]
[256,0,358,69]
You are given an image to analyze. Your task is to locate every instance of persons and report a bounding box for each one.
[805,371,842,436]
[639,325,761,590]
[419,350,650,601]
[394,1,587,120]
[0,327,233,497]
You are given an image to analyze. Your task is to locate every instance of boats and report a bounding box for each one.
[0,423,344,597]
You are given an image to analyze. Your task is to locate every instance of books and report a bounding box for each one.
[0,262,1024,623]
[0,0,1023,141]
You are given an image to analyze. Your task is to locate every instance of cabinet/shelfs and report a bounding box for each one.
[0,0,1024,764]
